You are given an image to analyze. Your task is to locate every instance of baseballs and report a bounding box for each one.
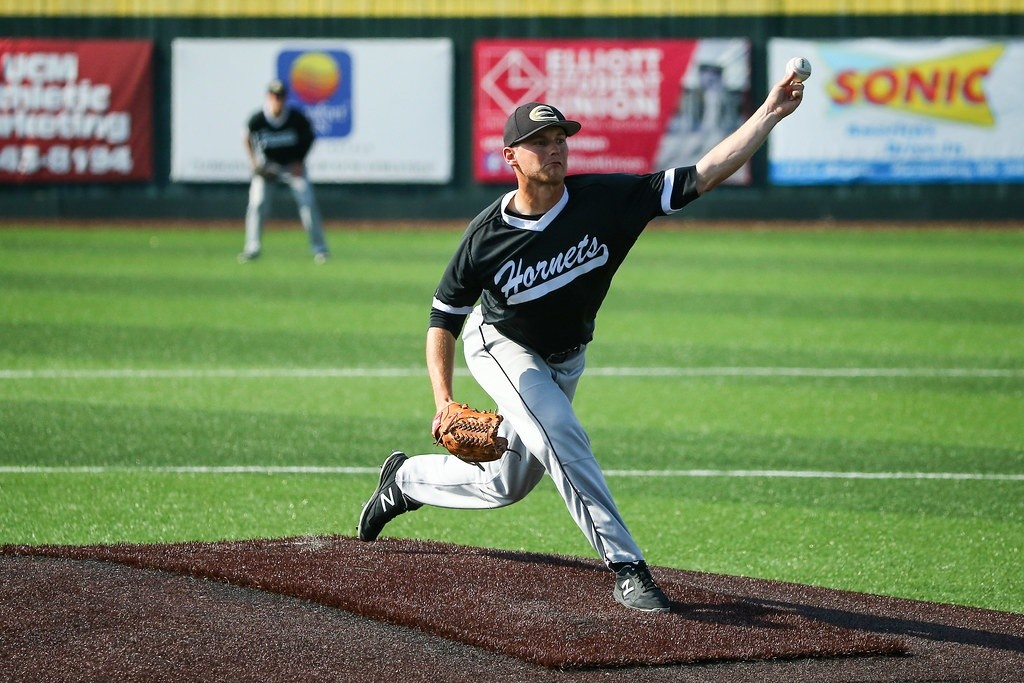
[785,57,812,83]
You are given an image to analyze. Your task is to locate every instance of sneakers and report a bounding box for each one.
[614,562,671,613]
[356,450,424,543]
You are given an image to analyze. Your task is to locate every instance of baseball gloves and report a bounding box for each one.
[431,402,509,466]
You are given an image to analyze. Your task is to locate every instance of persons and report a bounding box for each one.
[357,71,804,613]
[654,63,751,182]
[243,79,329,258]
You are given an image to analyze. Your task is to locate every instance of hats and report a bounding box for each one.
[502,101,581,147]
[268,78,286,94]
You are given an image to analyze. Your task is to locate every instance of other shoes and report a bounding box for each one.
[314,252,329,264]
[238,251,262,261]
